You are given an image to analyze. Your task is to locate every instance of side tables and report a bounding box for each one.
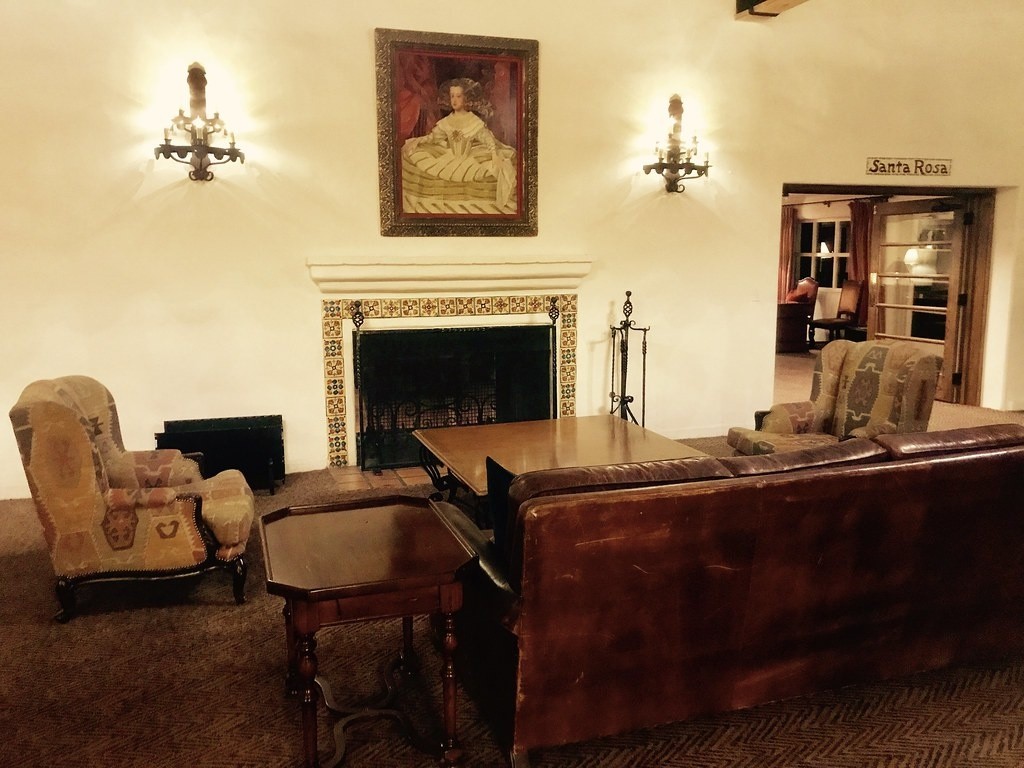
[258,495,480,768]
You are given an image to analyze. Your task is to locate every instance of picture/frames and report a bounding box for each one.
[374,27,539,235]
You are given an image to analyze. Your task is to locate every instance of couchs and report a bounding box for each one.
[428,421,1024,768]
[7,376,257,606]
[728,340,943,462]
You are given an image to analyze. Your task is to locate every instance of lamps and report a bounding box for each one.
[153,60,246,182]
[885,257,910,336]
[641,81,715,194]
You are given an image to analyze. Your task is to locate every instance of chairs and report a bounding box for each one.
[813,273,865,347]
[796,277,819,346]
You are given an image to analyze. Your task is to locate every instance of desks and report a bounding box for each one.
[413,414,714,527]
